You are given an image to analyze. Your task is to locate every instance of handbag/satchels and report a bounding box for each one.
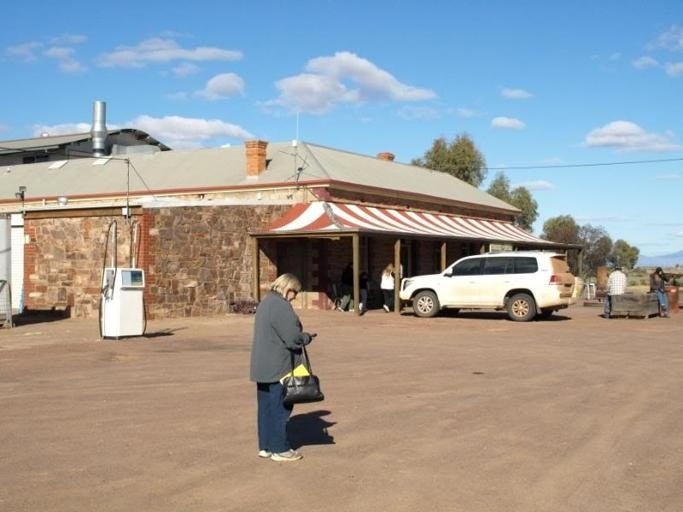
[278,334,324,406]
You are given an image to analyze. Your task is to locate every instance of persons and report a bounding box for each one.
[336,261,354,312]
[248,269,315,461]
[648,266,669,318]
[602,264,626,320]
[397,260,404,291]
[378,262,394,313]
[358,271,369,311]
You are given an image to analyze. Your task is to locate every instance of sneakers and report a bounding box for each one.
[256,447,303,464]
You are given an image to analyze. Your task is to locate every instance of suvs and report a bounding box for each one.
[397,250,575,322]
